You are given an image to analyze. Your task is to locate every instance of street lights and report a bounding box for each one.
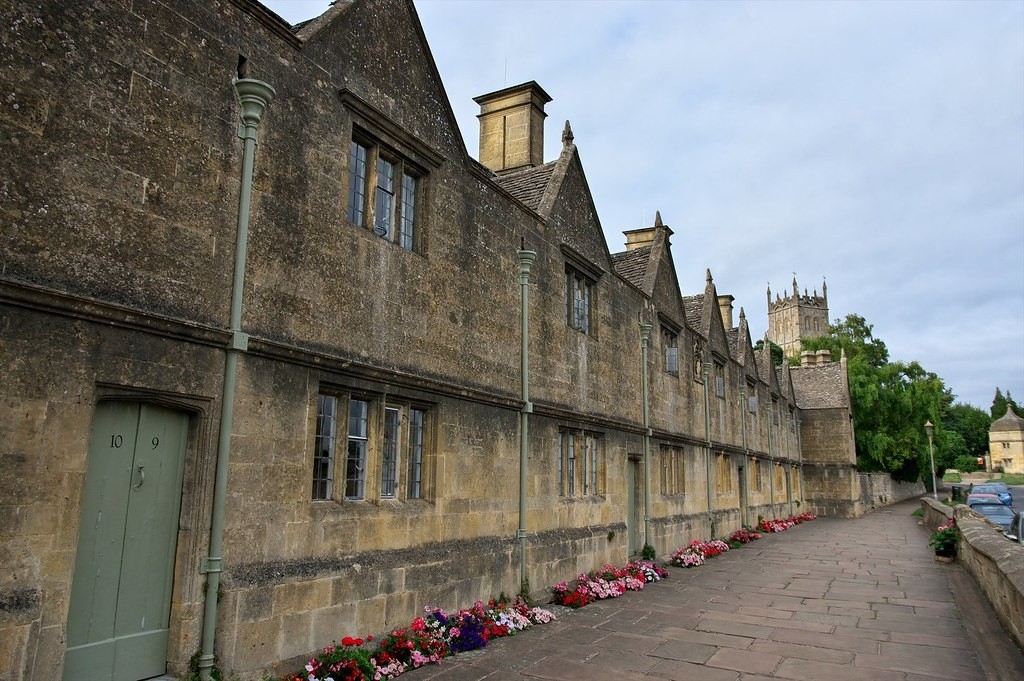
[924,420,938,501]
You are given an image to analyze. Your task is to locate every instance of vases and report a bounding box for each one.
[935,544,956,564]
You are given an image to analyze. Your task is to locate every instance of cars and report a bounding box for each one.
[970,503,1016,531]
[1003,513,1024,547]
[965,492,1015,512]
[967,481,1014,506]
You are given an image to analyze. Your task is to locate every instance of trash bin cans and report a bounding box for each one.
[952,485,962,501]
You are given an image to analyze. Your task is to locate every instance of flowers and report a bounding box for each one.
[928,519,966,552]
